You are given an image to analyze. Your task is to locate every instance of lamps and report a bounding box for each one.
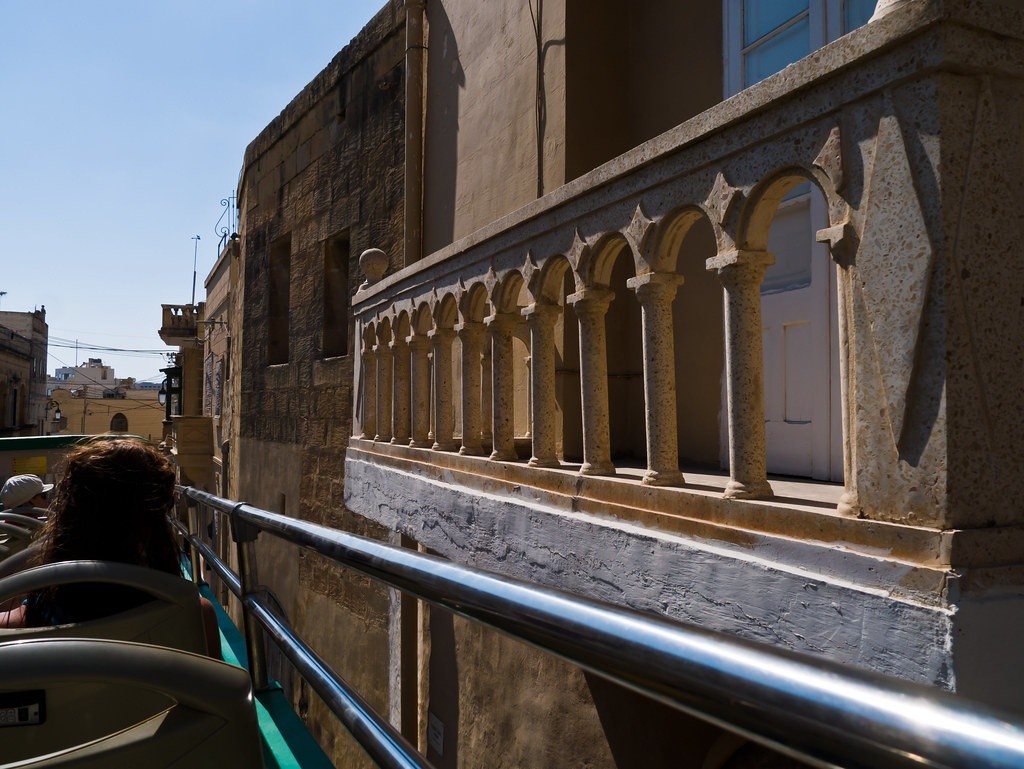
[46,400,61,420]
[158,378,167,406]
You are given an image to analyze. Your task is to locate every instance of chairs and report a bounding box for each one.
[0,506,266,769]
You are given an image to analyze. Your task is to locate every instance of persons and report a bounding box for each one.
[0,431,227,661]
[0,474,54,510]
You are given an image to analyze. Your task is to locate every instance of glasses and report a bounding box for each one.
[36,492,47,500]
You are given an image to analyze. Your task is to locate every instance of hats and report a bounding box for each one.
[0,474,54,510]
